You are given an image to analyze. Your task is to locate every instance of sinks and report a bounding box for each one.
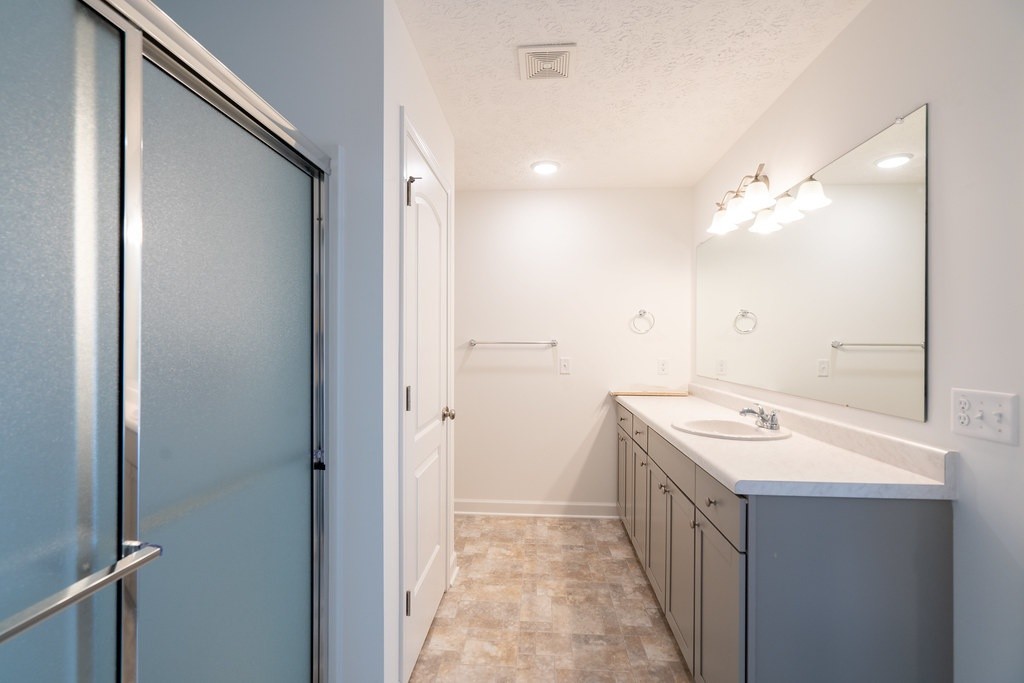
[669,417,793,442]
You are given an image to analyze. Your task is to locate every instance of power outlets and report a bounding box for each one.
[950,388,1020,446]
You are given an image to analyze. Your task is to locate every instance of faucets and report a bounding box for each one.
[737,404,780,430]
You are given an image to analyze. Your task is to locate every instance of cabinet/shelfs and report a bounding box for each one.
[615,400,956,683]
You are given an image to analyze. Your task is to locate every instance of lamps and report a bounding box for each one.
[706,163,775,236]
[748,176,830,236]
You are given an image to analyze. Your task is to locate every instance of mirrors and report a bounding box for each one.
[691,102,930,423]
